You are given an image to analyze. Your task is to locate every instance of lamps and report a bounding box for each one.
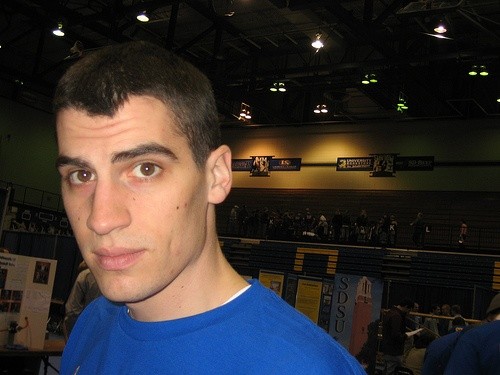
[51,9,490,120]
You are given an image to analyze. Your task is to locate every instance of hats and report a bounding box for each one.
[486,294,500,313]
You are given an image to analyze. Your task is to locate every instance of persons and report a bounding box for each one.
[54,40,368,375]
[410,212,424,248]
[227,203,400,248]
[421,295,494,375]
[64,268,103,344]
[382,300,484,350]
[444,293,500,375]
[377,296,416,375]
[458,219,468,248]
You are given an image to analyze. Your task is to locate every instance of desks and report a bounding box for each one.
[0,339,67,375]
